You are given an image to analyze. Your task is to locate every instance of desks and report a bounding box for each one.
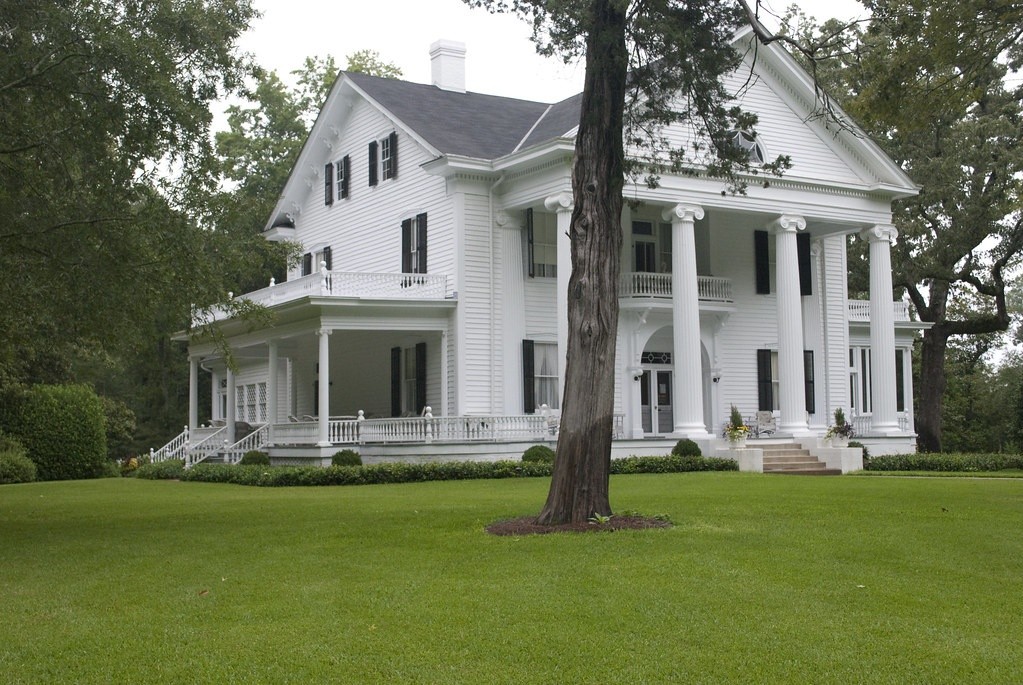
[314,415,359,423]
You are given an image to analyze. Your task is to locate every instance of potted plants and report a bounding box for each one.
[824,407,856,449]
[724,402,749,449]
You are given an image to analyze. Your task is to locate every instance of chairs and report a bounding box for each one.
[288,413,315,424]
[399,410,414,417]
[746,409,778,438]
[209,416,256,444]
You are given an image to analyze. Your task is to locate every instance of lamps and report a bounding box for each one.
[634,368,643,381]
[713,372,722,383]
